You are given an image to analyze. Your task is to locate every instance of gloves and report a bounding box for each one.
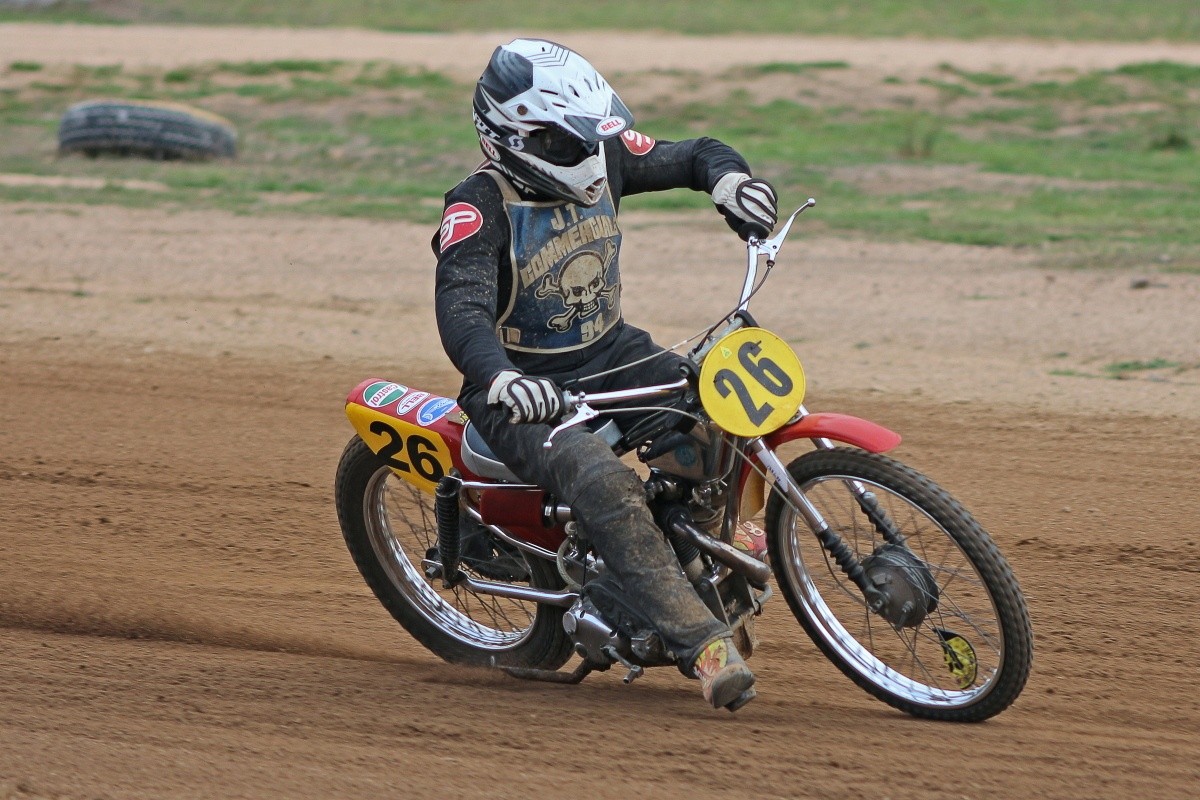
[710,172,778,243]
[486,372,565,424]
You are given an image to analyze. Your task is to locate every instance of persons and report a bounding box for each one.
[431,35,781,712]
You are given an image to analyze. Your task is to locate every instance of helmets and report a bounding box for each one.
[472,38,634,208]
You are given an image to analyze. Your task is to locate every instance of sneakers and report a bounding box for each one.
[700,515,770,557]
[691,641,757,712]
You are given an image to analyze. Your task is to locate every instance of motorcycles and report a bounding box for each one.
[333,196,1034,723]
